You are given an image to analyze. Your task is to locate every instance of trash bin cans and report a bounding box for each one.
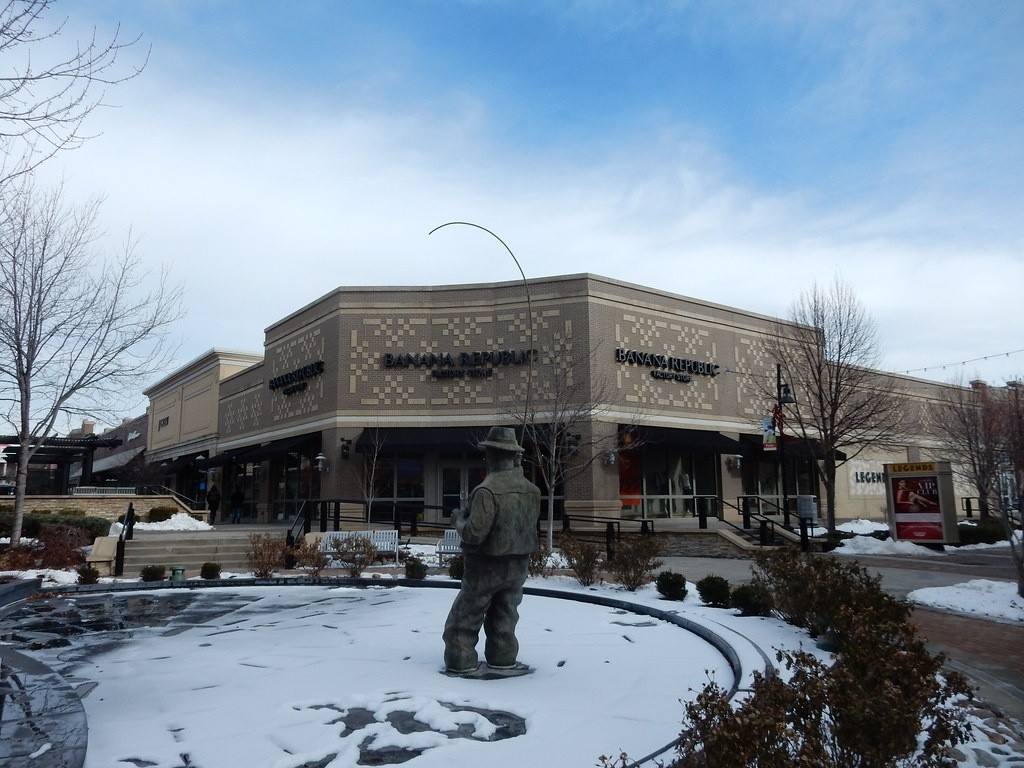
[797,495,818,526]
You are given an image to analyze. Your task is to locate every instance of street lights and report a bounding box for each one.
[776,364,798,526]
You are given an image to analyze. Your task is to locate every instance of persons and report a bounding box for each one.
[651,464,697,515]
[894,478,937,514]
[229,485,245,525]
[441,427,540,673]
[206,485,220,526]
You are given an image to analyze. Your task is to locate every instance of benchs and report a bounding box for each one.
[318,529,399,566]
[436,530,464,571]
